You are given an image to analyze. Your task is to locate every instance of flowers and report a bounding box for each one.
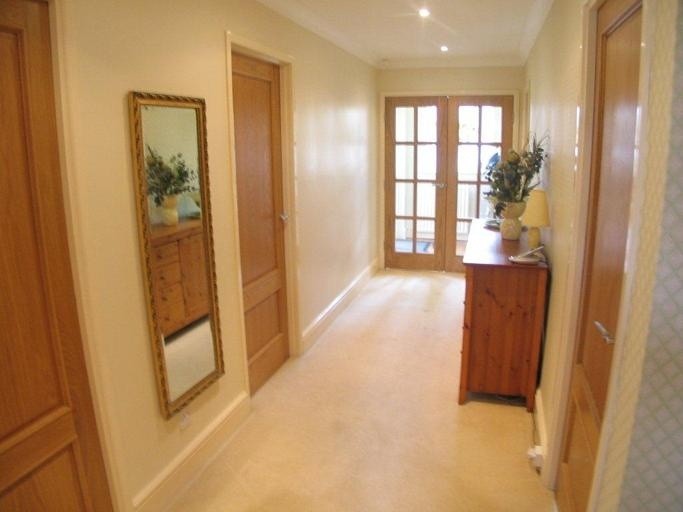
[485,128,548,216]
[144,143,198,205]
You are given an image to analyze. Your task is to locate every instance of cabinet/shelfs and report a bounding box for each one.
[145,220,211,341]
[459,217,550,413]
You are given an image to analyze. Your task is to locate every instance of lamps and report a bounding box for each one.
[517,187,550,251]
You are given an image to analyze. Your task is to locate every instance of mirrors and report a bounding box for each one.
[124,92,226,422]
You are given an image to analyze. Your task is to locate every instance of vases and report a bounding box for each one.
[493,199,524,240]
[156,191,181,226]
